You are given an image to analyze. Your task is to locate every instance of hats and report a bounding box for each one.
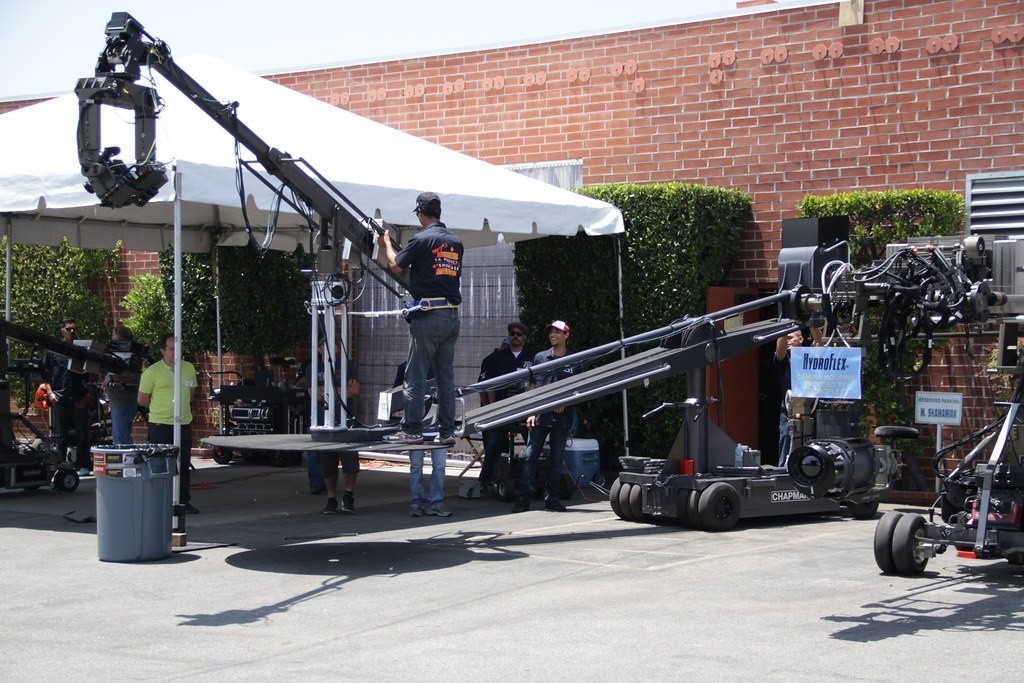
[545,320,570,334]
[502,337,510,344]
[412,192,441,212]
[508,322,526,335]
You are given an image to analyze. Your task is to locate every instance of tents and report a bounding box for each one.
[0,55,630,533]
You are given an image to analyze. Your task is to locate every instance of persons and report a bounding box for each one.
[774,326,823,465]
[294,331,362,513]
[41,316,96,476]
[408,364,452,516]
[137,333,201,515]
[100,325,144,445]
[478,320,580,512]
[379,192,464,445]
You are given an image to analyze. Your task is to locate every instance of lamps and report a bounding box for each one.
[294,232,305,258]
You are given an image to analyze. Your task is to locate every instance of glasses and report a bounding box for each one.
[509,331,522,337]
[65,326,79,331]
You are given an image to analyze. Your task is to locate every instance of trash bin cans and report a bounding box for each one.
[90,445,179,563]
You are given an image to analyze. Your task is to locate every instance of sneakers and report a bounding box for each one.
[409,507,424,517]
[433,435,456,445]
[544,500,567,512]
[323,500,338,514]
[511,500,531,512]
[382,430,424,444]
[180,502,200,514]
[342,494,356,513]
[424,505,452,517]
[78,468,90,476]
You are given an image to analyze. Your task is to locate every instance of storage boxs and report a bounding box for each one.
[0,451,50,489]
[526,438,600,489]
